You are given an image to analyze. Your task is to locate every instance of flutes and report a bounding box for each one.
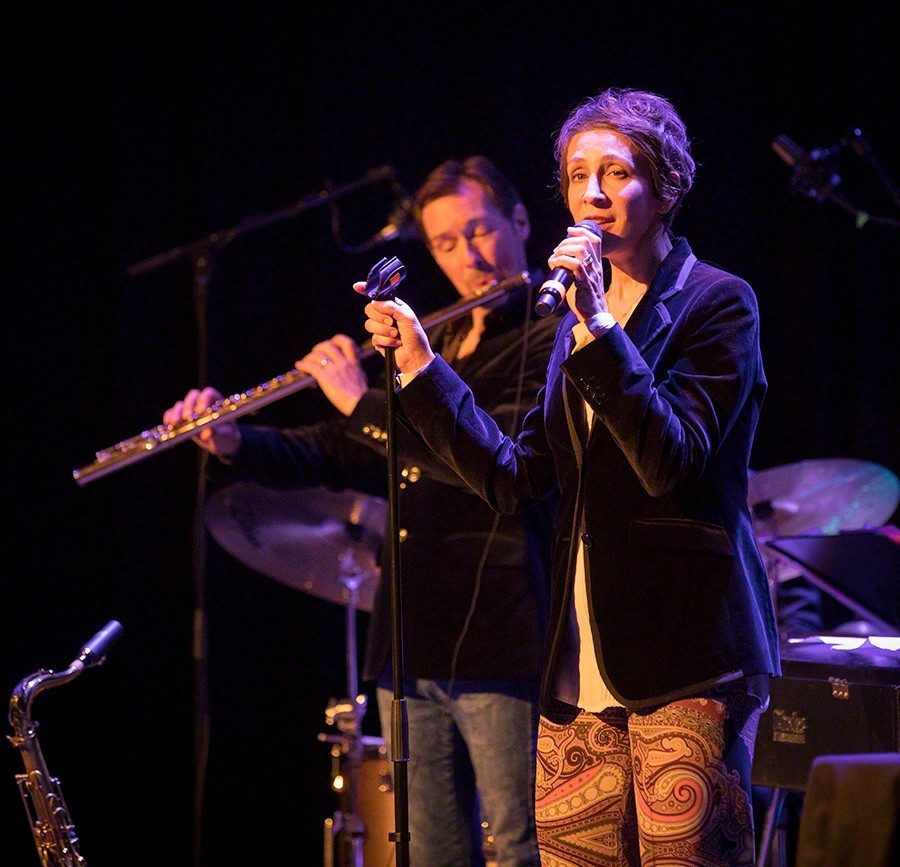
[69,269,537,486]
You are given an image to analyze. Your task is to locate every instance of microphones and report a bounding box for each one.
[534,220,603,318]
[374,202,417,245]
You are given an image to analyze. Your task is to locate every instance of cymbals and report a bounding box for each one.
[744,458,899,583]
[206,481,392,614]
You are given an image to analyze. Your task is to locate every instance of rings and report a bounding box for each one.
[320,358,332,367]
[581,253,594,266]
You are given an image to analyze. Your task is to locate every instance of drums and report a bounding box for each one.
[336,742,501,867]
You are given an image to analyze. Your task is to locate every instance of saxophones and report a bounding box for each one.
[5,618,127,867]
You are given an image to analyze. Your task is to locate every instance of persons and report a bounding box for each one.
[753,574,825,832]
[164,157,561,867]
[352,89,781,867]
